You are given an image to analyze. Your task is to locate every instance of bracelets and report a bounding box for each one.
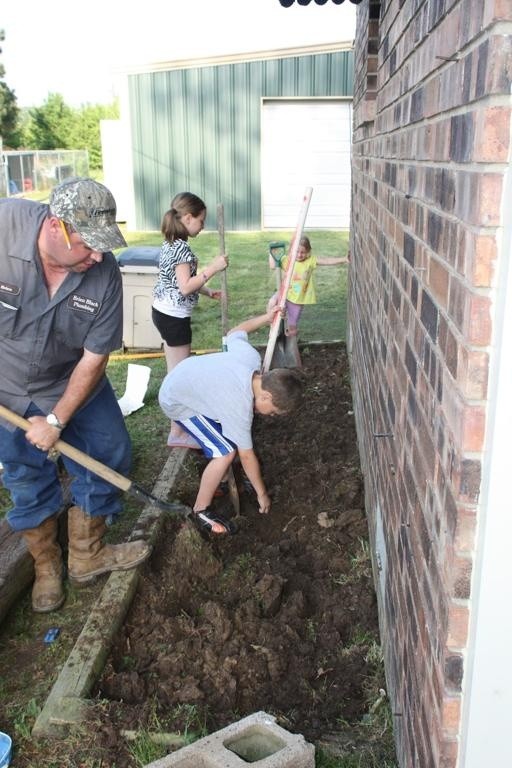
[201,271,209,284]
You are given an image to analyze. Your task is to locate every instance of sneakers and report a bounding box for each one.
[192,504,235,534]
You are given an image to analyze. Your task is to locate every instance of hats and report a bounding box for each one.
[49,177,128,252]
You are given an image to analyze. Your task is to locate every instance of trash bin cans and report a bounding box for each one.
[115,247,175,353]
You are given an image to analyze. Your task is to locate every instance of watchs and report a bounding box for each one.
[43,412,67,431]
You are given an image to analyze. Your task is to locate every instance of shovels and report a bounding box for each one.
[0,404,225,570]
[269,243,297,370]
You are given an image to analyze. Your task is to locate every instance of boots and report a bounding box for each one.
[16,514,67,613]
[67,505,153,587]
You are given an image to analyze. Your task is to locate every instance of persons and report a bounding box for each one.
[1,173,156,613]
[149,189,231,451]
[266,233,353,337]
[154,301,305,537]
